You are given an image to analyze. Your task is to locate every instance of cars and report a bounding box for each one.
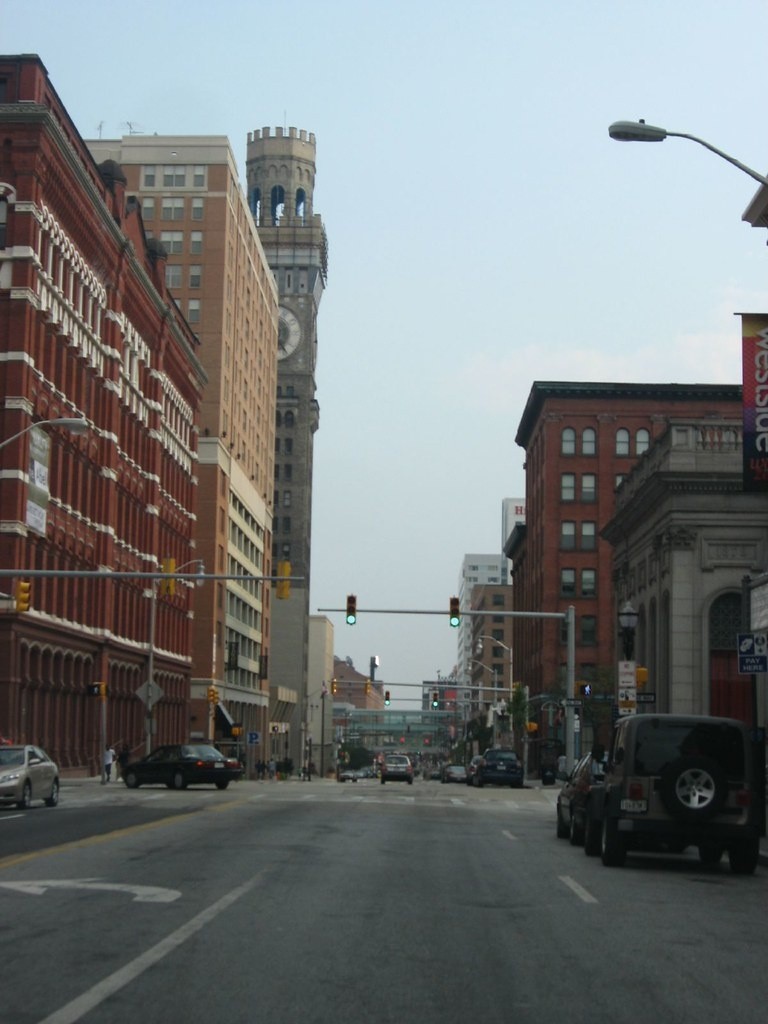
[380,755,414,784]
[121,744,241,789]
[556,749,609,845]
[431,749,525,789]
[0,744,60,810]
[338,768,376,782]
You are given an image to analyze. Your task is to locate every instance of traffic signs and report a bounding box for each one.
[637,692,655,704]
[558,698,584,707]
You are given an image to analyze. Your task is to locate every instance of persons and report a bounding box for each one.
[253,757,294,783]
[104,744,116,783]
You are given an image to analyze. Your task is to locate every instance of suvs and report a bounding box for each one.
[585,712,761,874]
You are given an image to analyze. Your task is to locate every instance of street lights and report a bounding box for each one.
[146,560,206,757]
[466,635,513,749]
[618,601,639,662]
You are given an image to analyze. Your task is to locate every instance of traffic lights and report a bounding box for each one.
[331,678,337,695]
[385,691,390,706]
[16,580,31,611]
[90,682,104,696]
[365,679,371,695]
[346,595,356,625]
[207,687,219,705]
[449,598,461,627]
[580,683,592,696]
[432,693,438,707]
